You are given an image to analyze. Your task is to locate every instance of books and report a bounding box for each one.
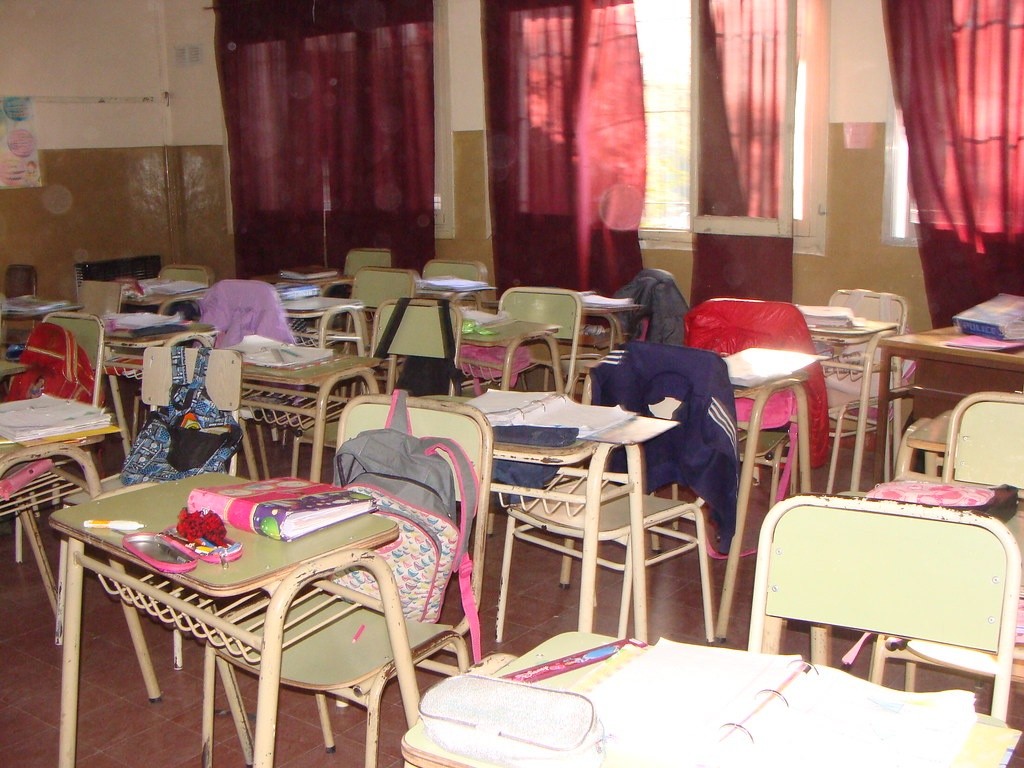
[0,391,122,450]
[138,278,208,296]
[795,303,853,328]
[463,388,637,440]
[864,480,1019,524]
[187,475,381,542]
[0,294,70,312]
[575,290,634,309]
[99,311,180,338]
[457,306,509,327]
[950,292,1023,341]
[574,637,980,765]
[272,282,319,302]
[278,265,338,279]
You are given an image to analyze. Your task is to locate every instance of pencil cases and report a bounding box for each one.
[122,524,243,574]
[493,424,579,447]
[418,673,605,768]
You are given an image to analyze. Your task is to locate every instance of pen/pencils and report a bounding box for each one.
[157,542,189,563]
[166,531,208,557]
[282,349,301,358]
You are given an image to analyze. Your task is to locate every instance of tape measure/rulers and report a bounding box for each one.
[271,347,285,364]
[498,637,649,685]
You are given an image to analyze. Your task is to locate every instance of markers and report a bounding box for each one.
[84,519,149,531]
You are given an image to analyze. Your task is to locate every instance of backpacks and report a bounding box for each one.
[461,344,530,395]
[845,289,915,418]
[4,322,95,404]
[371,296,461,396]
[332,389,481,653]
[120,346,243,487]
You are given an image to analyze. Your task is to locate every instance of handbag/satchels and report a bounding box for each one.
[736,387,796,430]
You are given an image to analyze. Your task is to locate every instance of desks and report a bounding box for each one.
[411,394,648,641]
[807,321,902,491]
[49,473,420,768]
[905,410,955,477]
[559,347,813,643]
[1,275,643,705]
[873,323,1024,491]
[808,489,1024,669]
[397,630,1023,768]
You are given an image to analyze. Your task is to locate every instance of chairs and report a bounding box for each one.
[10,311,107,565]
[493,340,731,645]
[497,286,583,395]
[76,281,123,318]
[199,394,493,768]
[5,264,37,300]
[368,298,465,399]
[865,391,1024,693]
[344,248,392,278]
[343,265,421,357]
[672,298,813,531]
[827,289,908,496]
[55,345,244,670]
[156,262,216,286]
[420,259,488,283]
[745,492,1024,724]
